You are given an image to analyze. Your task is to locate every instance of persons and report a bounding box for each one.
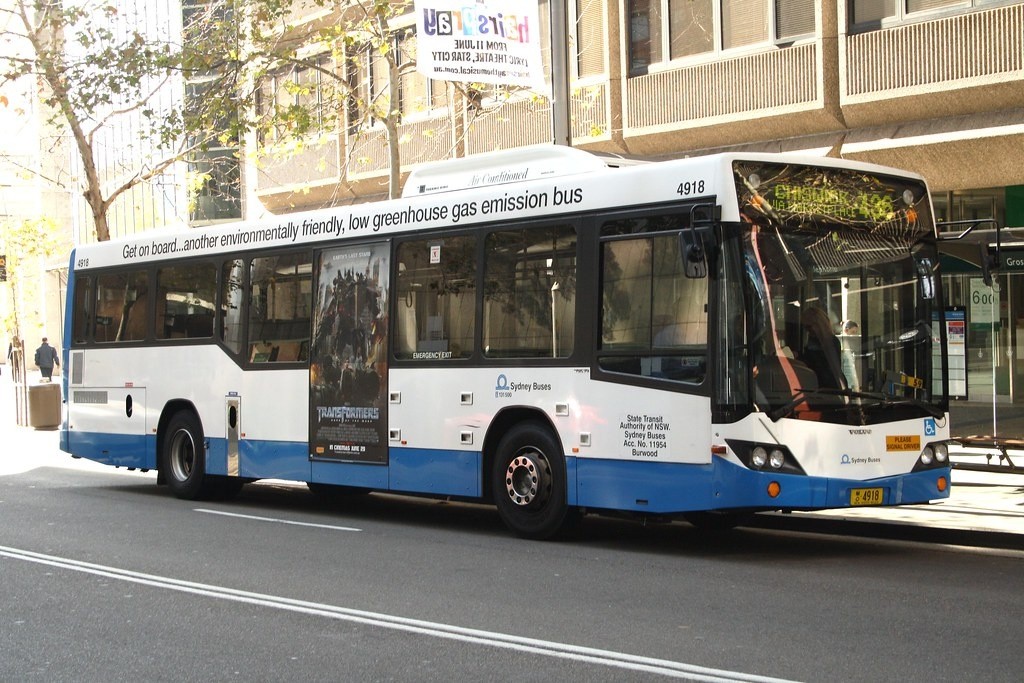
[34,337,59,381]
[8,336,21,382]
[839,320,861,405]
[653,296,699,346]
[792,307,843,394]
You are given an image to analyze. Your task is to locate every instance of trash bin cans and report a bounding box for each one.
[29,383,61,431]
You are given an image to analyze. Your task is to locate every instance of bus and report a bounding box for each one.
[59,141,1002,540]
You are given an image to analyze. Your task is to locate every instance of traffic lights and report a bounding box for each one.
[0,255,7,282]
